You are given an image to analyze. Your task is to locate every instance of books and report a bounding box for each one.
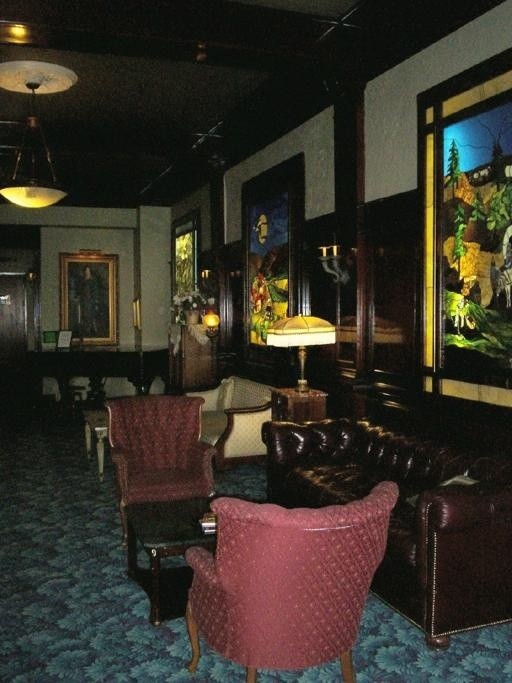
[198,517,216,535]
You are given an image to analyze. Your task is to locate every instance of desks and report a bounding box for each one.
[168,310,211,386]
[268,387,329,425]
[81,409,109,484]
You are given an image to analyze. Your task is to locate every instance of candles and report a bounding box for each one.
[202,272,205,278]
[206,270,208,278]
[332,245,337,256]
[323,247,326,257]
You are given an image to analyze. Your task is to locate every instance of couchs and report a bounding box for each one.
[261,415,511,651]
[184,376,277,482]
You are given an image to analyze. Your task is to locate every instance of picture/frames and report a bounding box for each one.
[60,252,119,347]
[171,205,201,324]
[233,152,305,368]
[411,48,511,422]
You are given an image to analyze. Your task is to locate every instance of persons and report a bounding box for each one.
[74,264,101,337]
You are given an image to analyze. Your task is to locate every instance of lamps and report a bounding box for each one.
[0,74,68,208]
[266,313,337,393]
[202,307,220,389]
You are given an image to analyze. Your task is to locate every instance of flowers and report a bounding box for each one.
[170,291,207,325]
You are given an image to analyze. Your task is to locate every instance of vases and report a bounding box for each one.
[183,309,200,324]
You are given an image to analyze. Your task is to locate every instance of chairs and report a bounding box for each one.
[184,479,400,683]
[104,395,220,549]
